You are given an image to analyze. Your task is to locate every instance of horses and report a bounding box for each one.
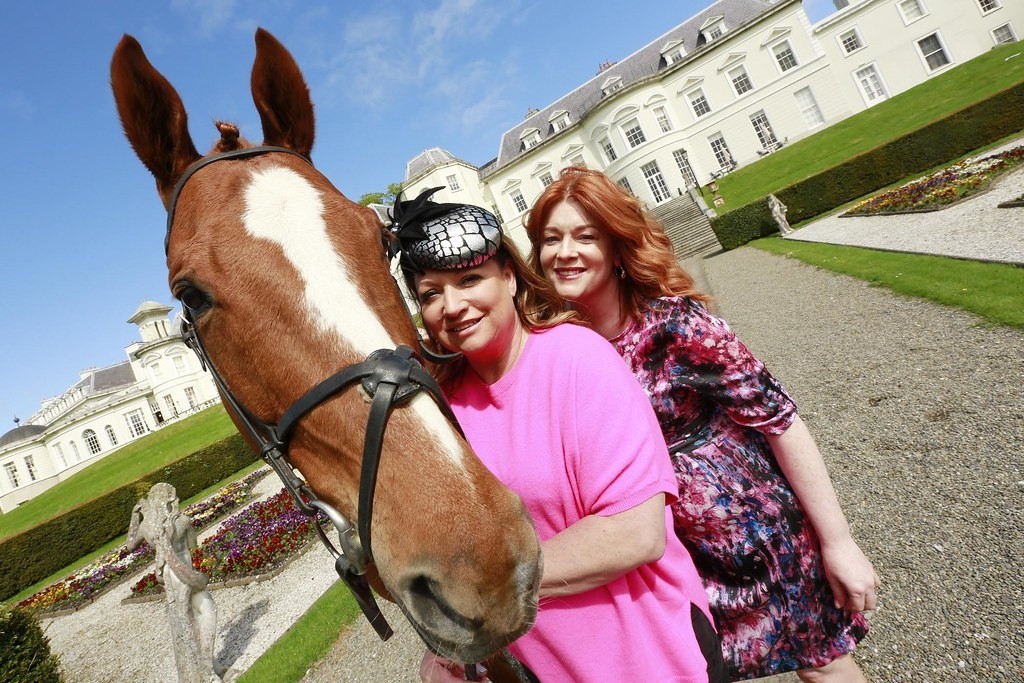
[111,27,544,664]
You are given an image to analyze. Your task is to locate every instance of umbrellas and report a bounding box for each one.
[759,123,775,153]
[719,144,733,169]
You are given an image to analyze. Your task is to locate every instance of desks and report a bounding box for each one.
[716,165,730,177]
[764,143,776,153]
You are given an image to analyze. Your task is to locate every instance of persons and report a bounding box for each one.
[380,185,730,683]
[520,164,882,683]
[765,191,795,233]
[125,498,223,683]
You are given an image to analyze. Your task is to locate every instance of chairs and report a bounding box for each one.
[775,142,783,150]
[709,172,720,180]
[756,150,770,157]
[730,161,738,173]
